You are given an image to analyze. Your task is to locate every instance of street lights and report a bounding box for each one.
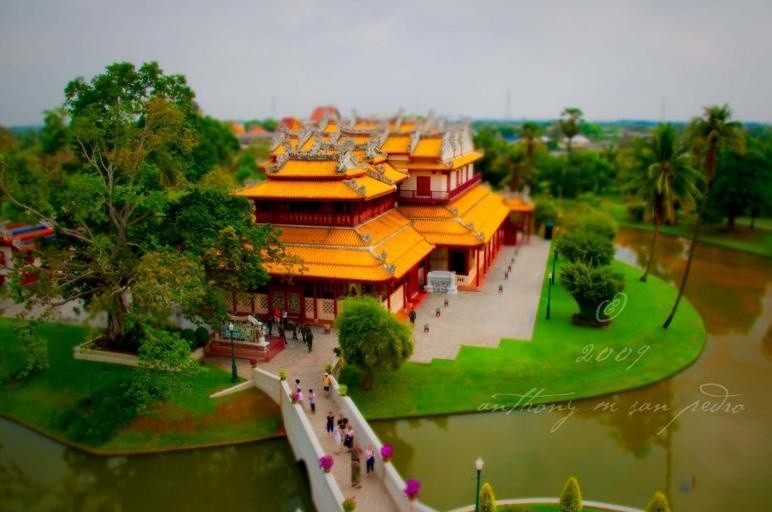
[474,456,485,512]
[229,321,240,383]
[545,272,553,320]
